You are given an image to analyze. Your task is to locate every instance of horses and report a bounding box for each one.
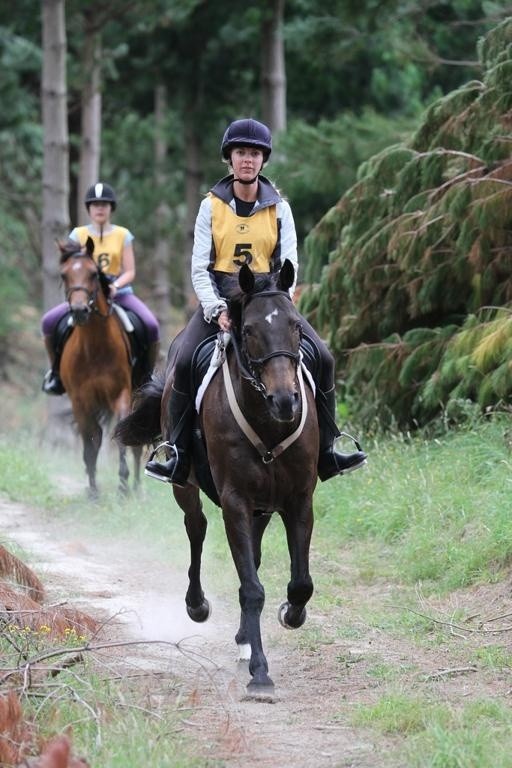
[47,231,146,500]
[114,257,325,695]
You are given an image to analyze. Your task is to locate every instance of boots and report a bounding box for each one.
[41,335,62,392]
[316,383,369,483]
[149,342,162,374]
[144,384,195,489]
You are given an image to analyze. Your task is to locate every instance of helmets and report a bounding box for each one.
[84,182,118,212]
[221,118,272,163]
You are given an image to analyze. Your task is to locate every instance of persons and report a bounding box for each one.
[40,182,161,395]
[145,119,368,489]
